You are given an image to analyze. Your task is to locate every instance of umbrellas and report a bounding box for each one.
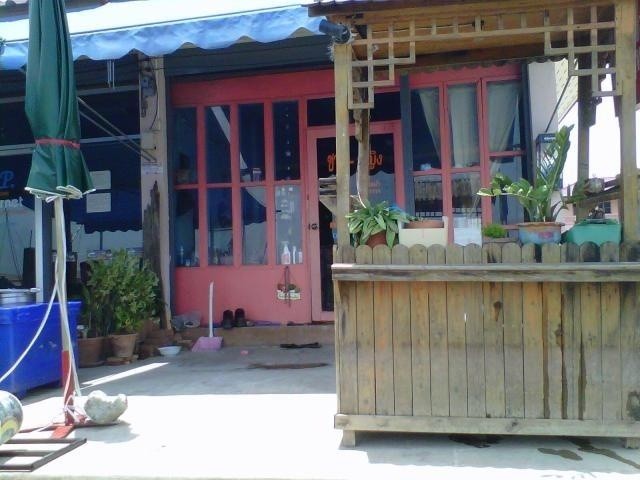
[25,0,96,422]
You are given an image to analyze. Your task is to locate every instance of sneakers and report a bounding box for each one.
[222,308,246,329]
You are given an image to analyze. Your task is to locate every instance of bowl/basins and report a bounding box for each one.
[158,346,182,357]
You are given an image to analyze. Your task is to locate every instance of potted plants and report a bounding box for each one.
[346,192,407,253]
[65,243,160,364]
[474,122,577,244]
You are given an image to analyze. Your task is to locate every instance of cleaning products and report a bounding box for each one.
[280,241,291,264]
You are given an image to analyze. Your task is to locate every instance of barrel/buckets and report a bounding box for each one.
[0,287,41,307]
